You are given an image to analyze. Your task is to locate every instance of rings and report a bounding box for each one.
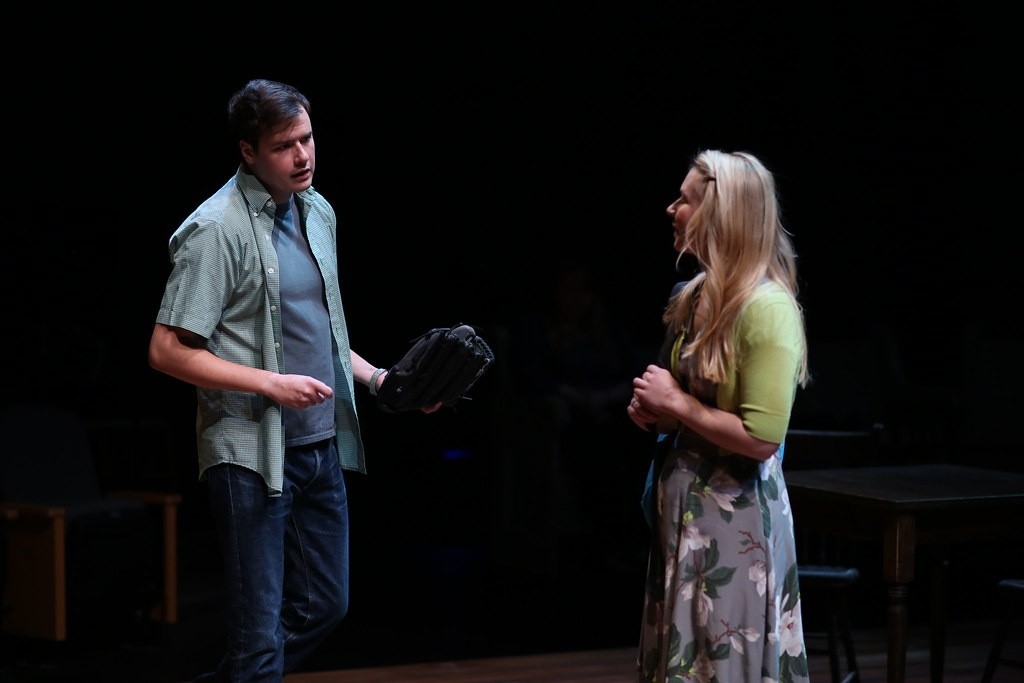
[631,400,637,406]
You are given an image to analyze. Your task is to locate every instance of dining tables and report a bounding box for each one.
[779,465,1024,683]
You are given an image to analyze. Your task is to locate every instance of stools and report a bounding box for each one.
[795,564,868,683]
[978,579,1024,683]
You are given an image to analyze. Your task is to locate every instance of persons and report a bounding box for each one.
[150,79,442,683]
[626,151,809,683]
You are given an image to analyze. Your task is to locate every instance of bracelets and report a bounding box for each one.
[370,369,385,396]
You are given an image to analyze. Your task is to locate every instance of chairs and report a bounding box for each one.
[0,404,184,642]
